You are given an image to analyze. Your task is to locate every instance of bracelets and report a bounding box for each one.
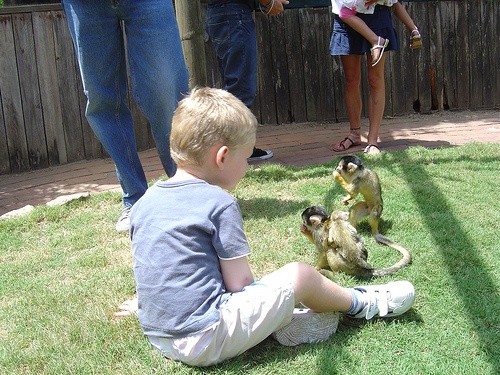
[265,0,274,15]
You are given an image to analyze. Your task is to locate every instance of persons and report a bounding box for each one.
[116,87,415,367]
[339,0,423,66]
[61,0,191,231]
[329,0,399,155]
[200,0,289,161]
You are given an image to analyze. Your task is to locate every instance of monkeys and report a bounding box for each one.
[331,154,412,278]
[298,205,374,282]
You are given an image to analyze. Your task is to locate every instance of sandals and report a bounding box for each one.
[363,143,381,156]
[332,133,362,152]
[370,36,389,67]
[409,26,422,49]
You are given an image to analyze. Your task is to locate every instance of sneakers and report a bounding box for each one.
[247,147,274,161]
[345,280,415,320]
[116,201,133,233]
[273,307,339,347]
